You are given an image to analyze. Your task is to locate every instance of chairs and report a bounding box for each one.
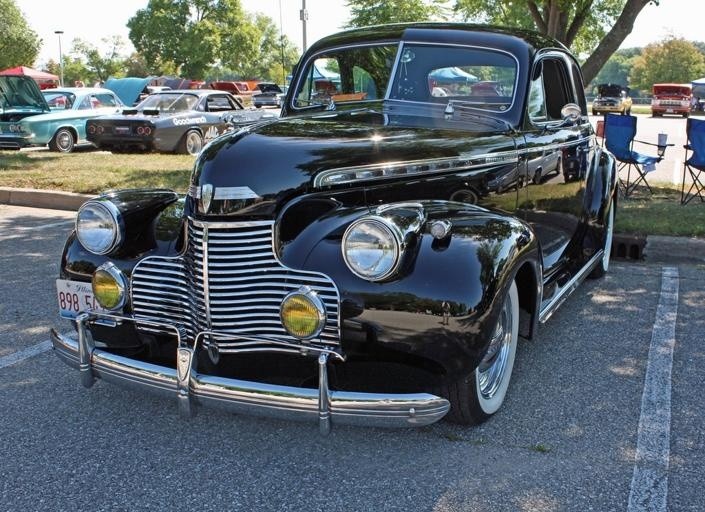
[681,114,705,208]
[601,112,663,201]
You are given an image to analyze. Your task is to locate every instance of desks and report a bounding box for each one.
[628,137,677,195]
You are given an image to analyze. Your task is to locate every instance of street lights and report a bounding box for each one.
[53,29,65,87]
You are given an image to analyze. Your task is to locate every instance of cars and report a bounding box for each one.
[136,78,193,105]
[647,84,698,118]
[252,83,290,108]
[211,81,253,105]
[0,74,158,154]
[469,79,506,97]
[311,79,342,99]
[592,83,633,117]
[85,89,279,156]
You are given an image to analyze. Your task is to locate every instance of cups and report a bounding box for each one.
[658,133,668,147]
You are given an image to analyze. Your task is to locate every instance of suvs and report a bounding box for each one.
[49,22,618,426]
